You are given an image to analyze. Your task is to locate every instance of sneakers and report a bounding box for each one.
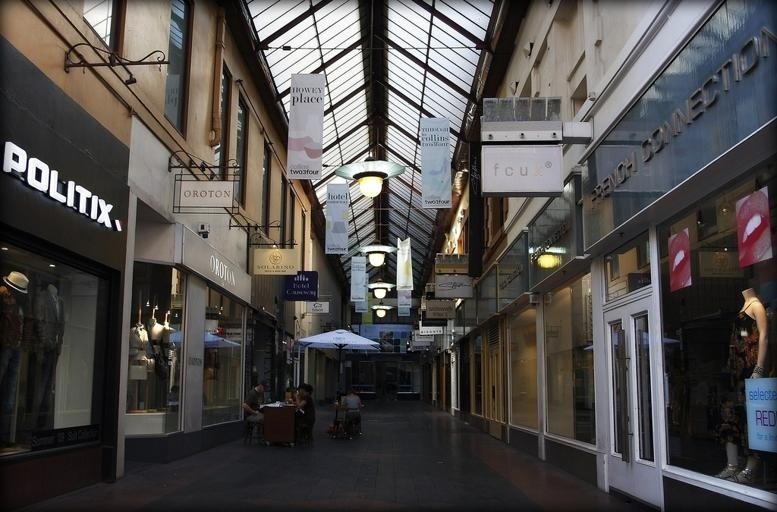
[0,447,20,457]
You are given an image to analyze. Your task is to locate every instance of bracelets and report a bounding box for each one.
[751,365,766,376]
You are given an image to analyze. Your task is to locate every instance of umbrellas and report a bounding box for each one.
[168,330,241,349]
[295,327,382,422]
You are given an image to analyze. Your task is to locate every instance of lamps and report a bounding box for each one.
[360,197,397,267]
[334,128,406,199]
[371,299,393,318]
[366,267,396,299]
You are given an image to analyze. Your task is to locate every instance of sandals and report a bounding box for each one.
[714,463,740,479]
[726,467,759,485]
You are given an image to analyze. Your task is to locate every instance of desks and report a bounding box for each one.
[329,404,364,422]
[263,403,297,448]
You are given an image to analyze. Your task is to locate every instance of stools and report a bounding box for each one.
[244,419,261,445]
[303,416,315,448]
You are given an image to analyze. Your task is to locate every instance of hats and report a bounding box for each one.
[2,271,30,294]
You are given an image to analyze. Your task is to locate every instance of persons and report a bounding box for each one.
[0,268,26,449]
[241,380,267,425]
[287,382,316,425]
[711,286,769,484]
[24,282,67,447]
[342,388,363,437]
[128,317,176,411]
